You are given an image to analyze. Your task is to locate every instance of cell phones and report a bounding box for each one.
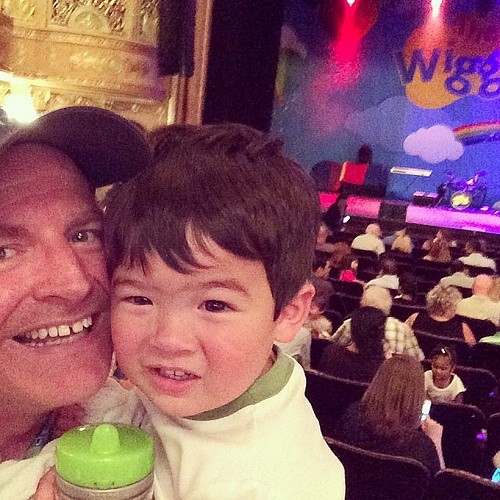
[420,399,432,420]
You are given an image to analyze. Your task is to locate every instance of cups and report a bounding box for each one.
[54,421,155,500]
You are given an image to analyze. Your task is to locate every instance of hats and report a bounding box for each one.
[0,106,152,190]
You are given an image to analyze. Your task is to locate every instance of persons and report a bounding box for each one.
[357,142,371,163]
[0,104,149,500]
[275,192,499,500]
[104,123,347,500]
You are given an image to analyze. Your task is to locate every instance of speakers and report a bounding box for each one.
[379,202,407,221]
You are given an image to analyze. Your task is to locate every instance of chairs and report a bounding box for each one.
[239,218,500,500]
